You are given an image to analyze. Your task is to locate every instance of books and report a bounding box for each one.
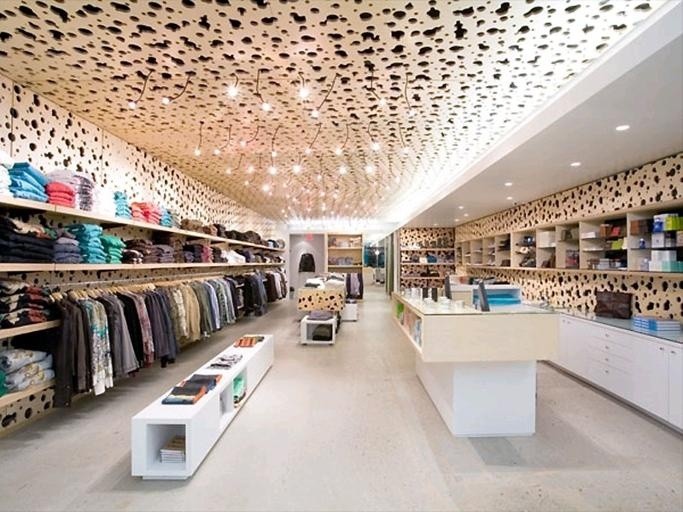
[632,315,681,331]
[160,435,185,462]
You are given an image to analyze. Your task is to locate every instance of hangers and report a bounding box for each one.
[49,280,127,301]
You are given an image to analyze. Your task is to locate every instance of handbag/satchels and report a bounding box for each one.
[594,290,634,320]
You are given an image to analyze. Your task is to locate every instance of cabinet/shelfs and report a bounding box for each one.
[399,246,454,290]
[325,233,365,303]
[130,333,274,480]
[389,287,559,437]
[0,193,285,436]
[553,306,683,433]
[454,198,683,278]
[297,273,357,345]
